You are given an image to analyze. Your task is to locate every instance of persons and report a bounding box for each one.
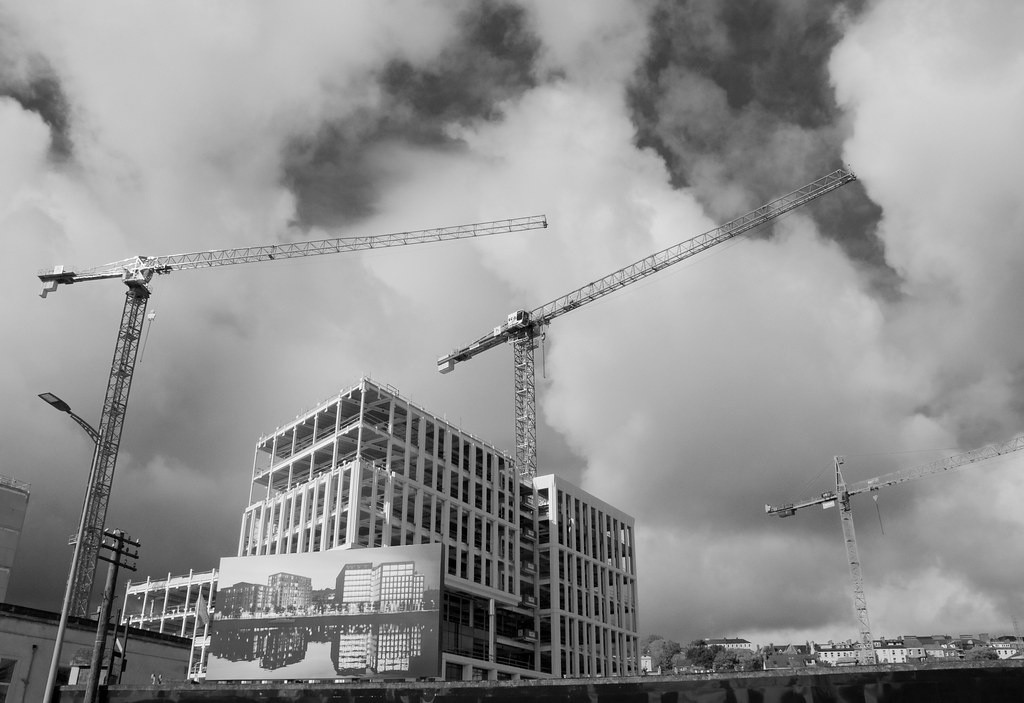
[150,674,163,685]
[855,660,859,665]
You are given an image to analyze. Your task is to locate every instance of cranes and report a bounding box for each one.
[436,161,868,481]
[37,210,550,619]
[765,433,1024,665]
